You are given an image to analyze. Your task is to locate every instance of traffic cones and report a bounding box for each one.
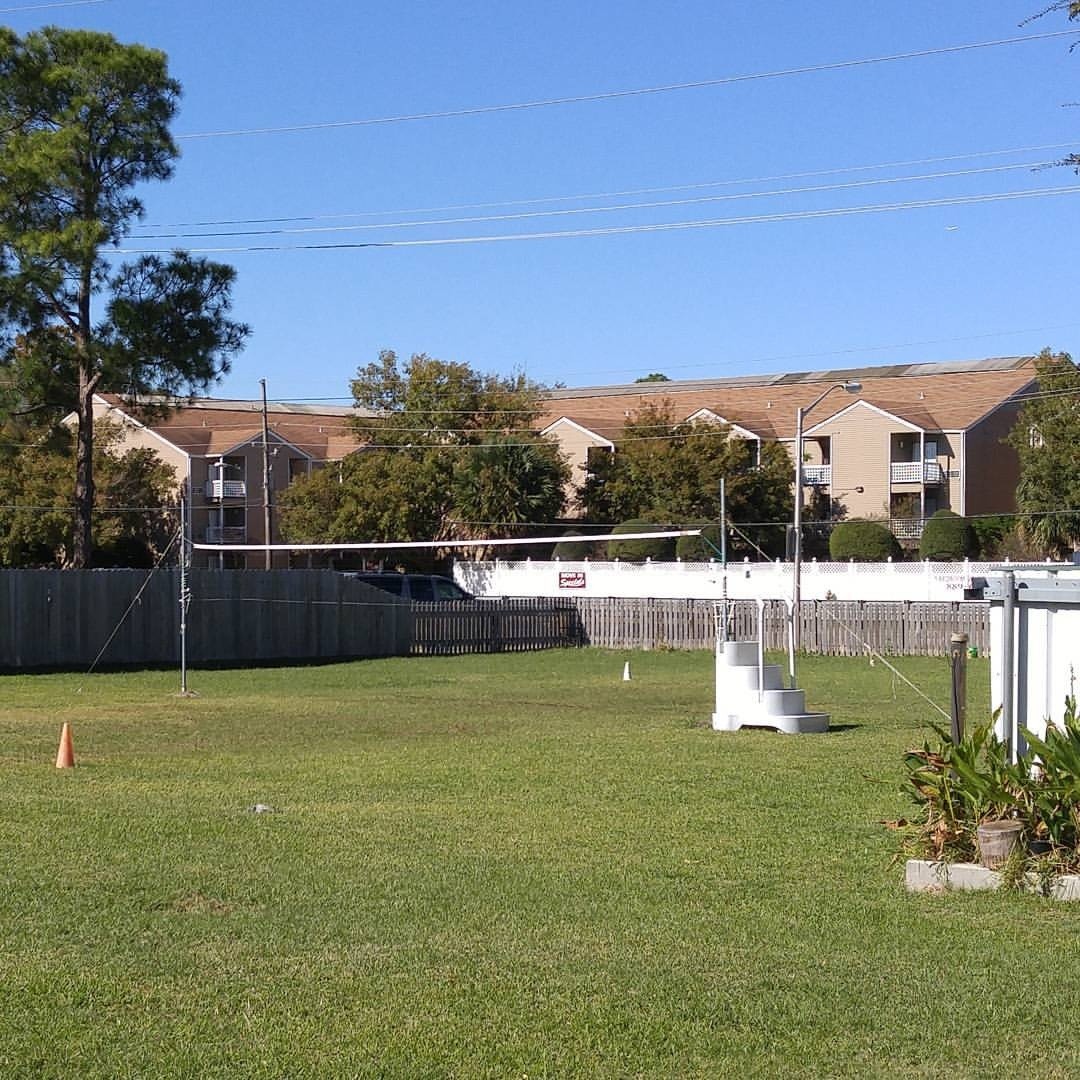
[621,661,632,683]
[53,720,76,769]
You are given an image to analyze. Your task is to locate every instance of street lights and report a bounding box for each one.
[794,382,864,646]
